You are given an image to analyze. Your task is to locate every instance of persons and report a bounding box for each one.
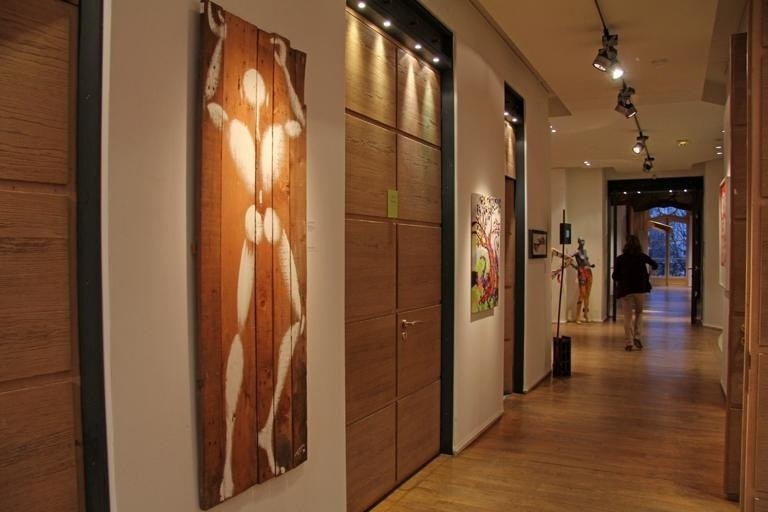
[611,234,659,352]
[568,236,595,325]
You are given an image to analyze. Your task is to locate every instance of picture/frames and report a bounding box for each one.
[529,229,548,258]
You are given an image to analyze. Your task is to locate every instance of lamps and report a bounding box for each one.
[590,31,655,175]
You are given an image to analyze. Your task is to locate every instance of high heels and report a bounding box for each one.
[624,338,644,351]
